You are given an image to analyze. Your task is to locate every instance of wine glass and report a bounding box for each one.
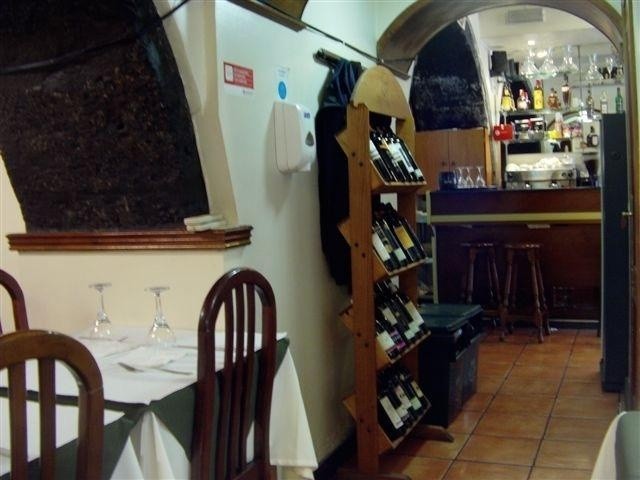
[145,285,176,360]
[584,50,616,81]
[561,86,570,111]
[456,165,486,188]
[89,282,113,339]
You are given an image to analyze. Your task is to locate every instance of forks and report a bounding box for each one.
[118,362,193,377]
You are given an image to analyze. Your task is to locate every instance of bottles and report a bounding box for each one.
[370,125,424,182]
[375,276,429,358]
[440,161,457,188]
[586,126,599,147]
[501,79,559,111]
[522,41,578,75]
[372,202,427,272]
[377,361,428,439]
[586,86,624,119]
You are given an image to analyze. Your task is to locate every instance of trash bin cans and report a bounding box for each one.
[416,303,483,429]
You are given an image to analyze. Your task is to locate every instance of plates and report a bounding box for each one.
[123,346,186,368]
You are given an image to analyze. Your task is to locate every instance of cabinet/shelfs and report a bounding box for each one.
[334,62,443,473]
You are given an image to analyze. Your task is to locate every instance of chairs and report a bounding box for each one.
[0,329,106,478]
[194,258,280,480]
[0,267,31,333]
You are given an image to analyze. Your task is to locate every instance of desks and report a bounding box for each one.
[0,314,290,478]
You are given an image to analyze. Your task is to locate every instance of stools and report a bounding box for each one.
[462,238,552,343]
